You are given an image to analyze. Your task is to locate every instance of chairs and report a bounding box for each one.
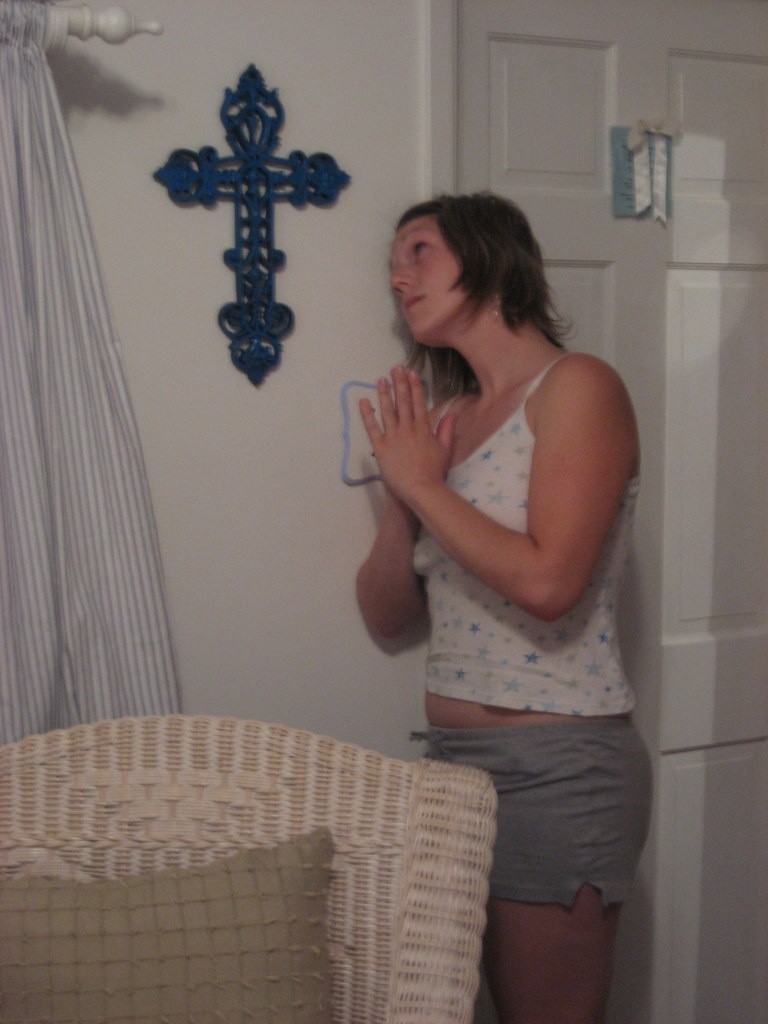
[0,712,499,1024]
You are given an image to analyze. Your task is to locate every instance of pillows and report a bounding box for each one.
[0,824,336,1024]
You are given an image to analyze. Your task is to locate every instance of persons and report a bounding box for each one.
[353,191,657,1024]
